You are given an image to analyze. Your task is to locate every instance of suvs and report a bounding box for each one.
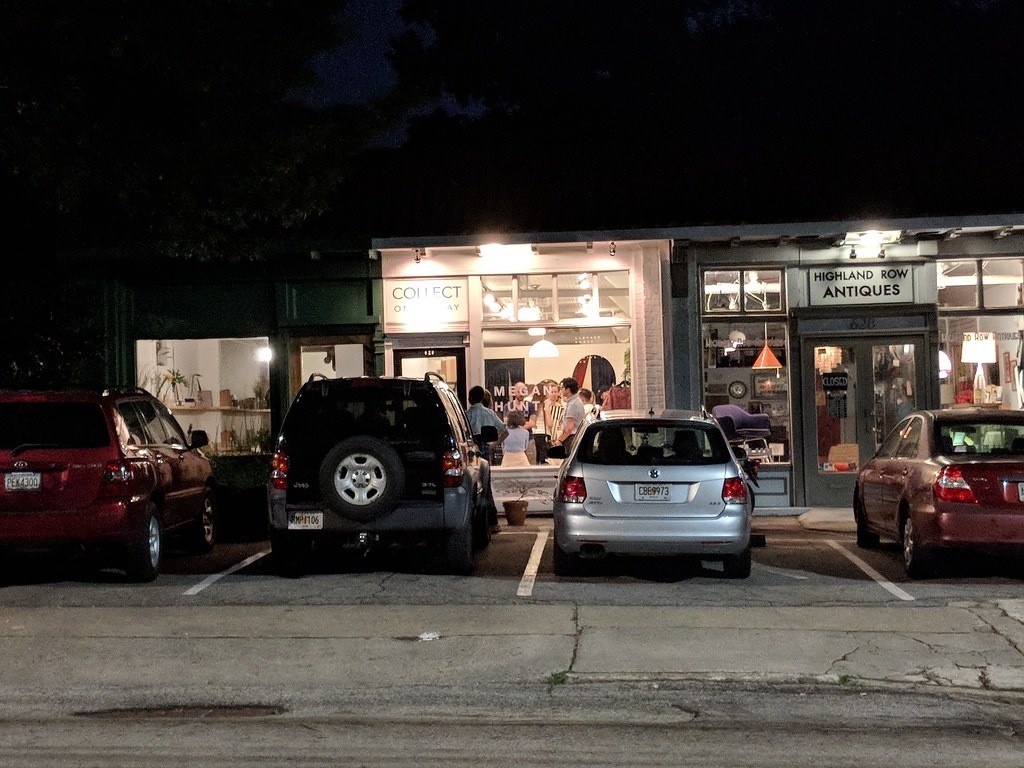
[269,372,499,569]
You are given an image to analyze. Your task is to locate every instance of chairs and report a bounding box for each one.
[358,397,391,427]
[593,425,632,459]
[671,430,703,461]
[399,406,442,432]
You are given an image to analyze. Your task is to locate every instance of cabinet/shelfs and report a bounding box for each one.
[169,406,272,455]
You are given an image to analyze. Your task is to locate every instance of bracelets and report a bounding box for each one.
[559,440,563,443]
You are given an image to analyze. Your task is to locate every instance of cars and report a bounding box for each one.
[853,408,1024,579]
[0,385,217,581]
[553,405,756,575]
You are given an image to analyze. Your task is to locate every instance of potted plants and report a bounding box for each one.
[195,375,272,453]
[503,484,529,527]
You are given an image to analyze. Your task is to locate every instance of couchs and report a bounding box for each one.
[712,404,772,438]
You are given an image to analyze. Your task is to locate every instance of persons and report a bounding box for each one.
[554,377,585,455]
[501,413,530,467]
[532,381,601,466]
[504,382,536,431]
[464,386,509,461]
[482,388,493,410]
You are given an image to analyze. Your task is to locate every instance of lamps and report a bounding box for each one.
[609,241,617,256]
[751,321,783,369]
[480,271,600,336]
[878,247,885,259]
[960,332,997,404]
[529,339,559,357]
[849,244,856,259]
[414,248,422,264]
